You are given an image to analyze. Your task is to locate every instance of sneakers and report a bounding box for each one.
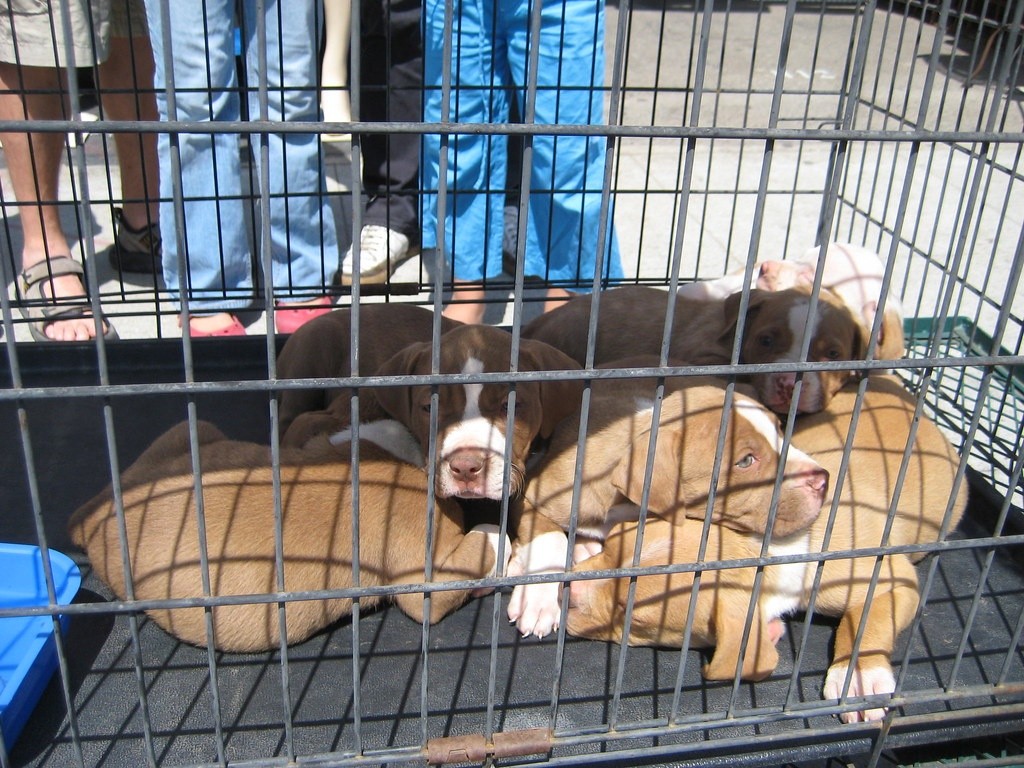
[342,224,410,286]
[503,205,519,278]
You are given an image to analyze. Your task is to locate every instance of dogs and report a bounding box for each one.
[65,241,970,725]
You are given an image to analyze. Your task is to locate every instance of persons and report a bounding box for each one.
[141,0,622,337]
[0,0,163,344]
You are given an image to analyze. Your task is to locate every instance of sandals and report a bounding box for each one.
[14,255,120,342]
[109,207,163,273]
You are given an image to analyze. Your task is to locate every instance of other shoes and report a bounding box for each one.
[178,311,246,337]
[276,296,336,333]
